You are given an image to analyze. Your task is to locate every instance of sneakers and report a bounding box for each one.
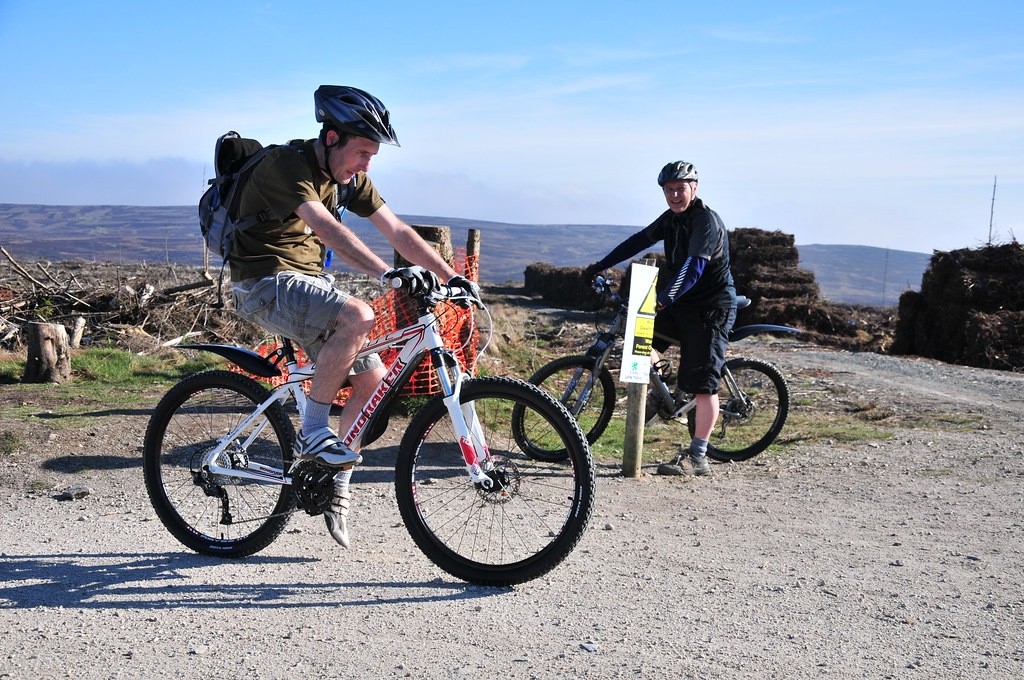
[656,449,713,476]
[291,428,359,464]
[322,475,352,552]
[618,384,670,408]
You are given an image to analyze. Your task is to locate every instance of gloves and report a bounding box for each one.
[447,274,486,310]
[581,269,612,292]
[382,264,444,306]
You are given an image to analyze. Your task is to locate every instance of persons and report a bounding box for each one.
[583,161,736,476]
[232,84,484,548]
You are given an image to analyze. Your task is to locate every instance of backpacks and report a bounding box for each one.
[197,129,322,309]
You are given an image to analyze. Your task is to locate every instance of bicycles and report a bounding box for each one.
[511,270,801,463]
[142,276,597,588]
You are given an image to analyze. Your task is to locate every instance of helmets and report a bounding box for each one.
[313,83,402,148]
[656,160,699,186]
